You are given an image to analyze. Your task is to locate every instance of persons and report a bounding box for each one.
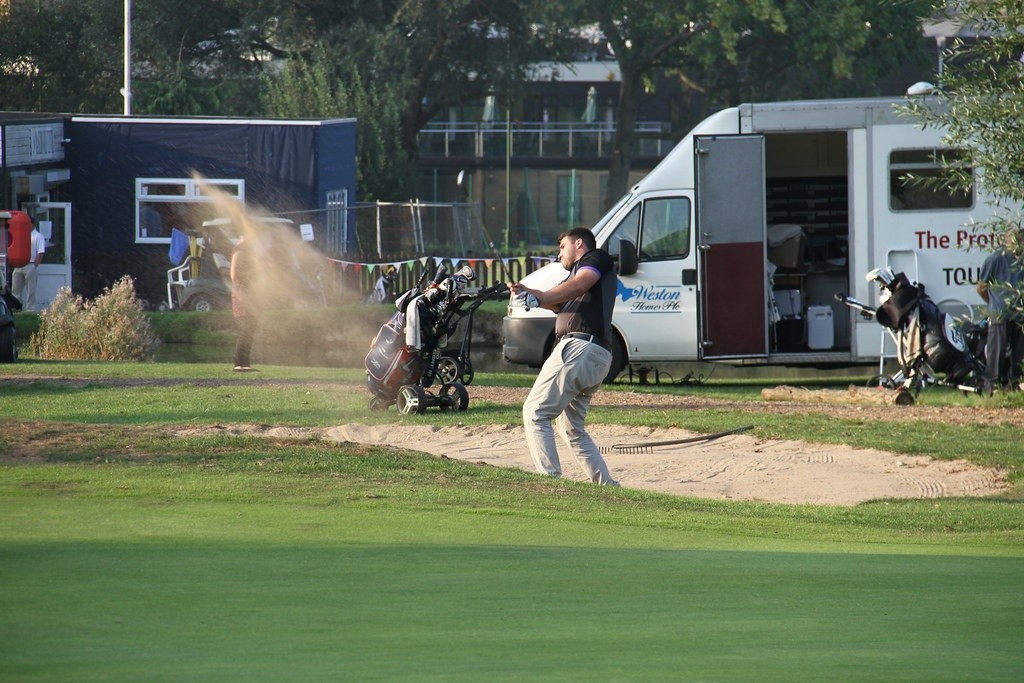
[507,227,622,487]
[11,216,45,309]
[366,266,398,303]
[230,235,263,372]
[977,227,1024,394]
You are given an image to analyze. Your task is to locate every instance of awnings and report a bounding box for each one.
[466,60,623,84]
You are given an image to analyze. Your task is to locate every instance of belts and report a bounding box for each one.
[563,334,611,353]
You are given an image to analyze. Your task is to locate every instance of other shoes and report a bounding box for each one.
[233,367,261,373]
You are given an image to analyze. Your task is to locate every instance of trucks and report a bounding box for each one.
[503,92,1024,385]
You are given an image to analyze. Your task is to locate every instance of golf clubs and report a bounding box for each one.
[865,266,903,304]
[456,168,531,312]
[400,255,479,335]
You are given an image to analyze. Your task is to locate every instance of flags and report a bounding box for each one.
[167,227,189,266]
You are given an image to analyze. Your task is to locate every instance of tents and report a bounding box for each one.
[921,7,1024,91]
